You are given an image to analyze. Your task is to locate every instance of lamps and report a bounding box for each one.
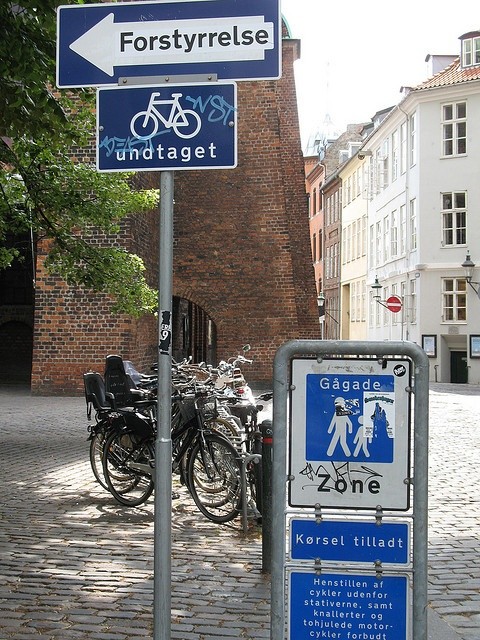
[459,249,476,283]
[371,274,383,301]
[317,292,325,307]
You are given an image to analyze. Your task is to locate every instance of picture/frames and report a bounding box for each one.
[421,333,437,358]
[469,334,480,358]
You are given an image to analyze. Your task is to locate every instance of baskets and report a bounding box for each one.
[177,399,215,421]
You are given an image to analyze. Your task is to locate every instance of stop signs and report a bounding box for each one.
[386,297,402,313]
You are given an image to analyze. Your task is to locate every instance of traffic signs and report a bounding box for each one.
[59,0,284,89]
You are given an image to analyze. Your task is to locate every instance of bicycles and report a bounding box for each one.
[77,341,272,524]
